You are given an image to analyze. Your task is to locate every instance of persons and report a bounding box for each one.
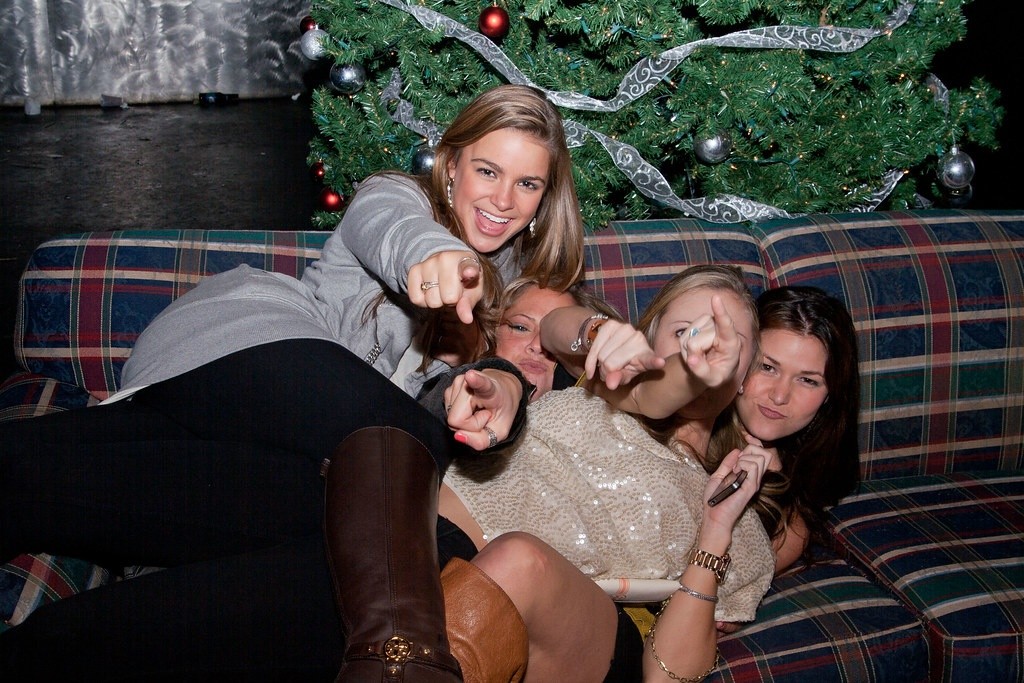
[0,265,861,683]
[1,83,584,683]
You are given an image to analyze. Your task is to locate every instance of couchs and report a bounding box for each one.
[0,211,1024,683]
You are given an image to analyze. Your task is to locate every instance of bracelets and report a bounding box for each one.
[587,320,607,350]
[571,314,608,352]
[681,585,720,602]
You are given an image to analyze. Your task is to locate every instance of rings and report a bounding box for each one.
[690,328,701,338]
[421,281,440,293]
[484,427,498,448]
[457,257,481,282]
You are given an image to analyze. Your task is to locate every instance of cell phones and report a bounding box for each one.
[708,469,748,506]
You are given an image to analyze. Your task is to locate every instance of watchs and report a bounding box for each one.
[688,548,732,582]
